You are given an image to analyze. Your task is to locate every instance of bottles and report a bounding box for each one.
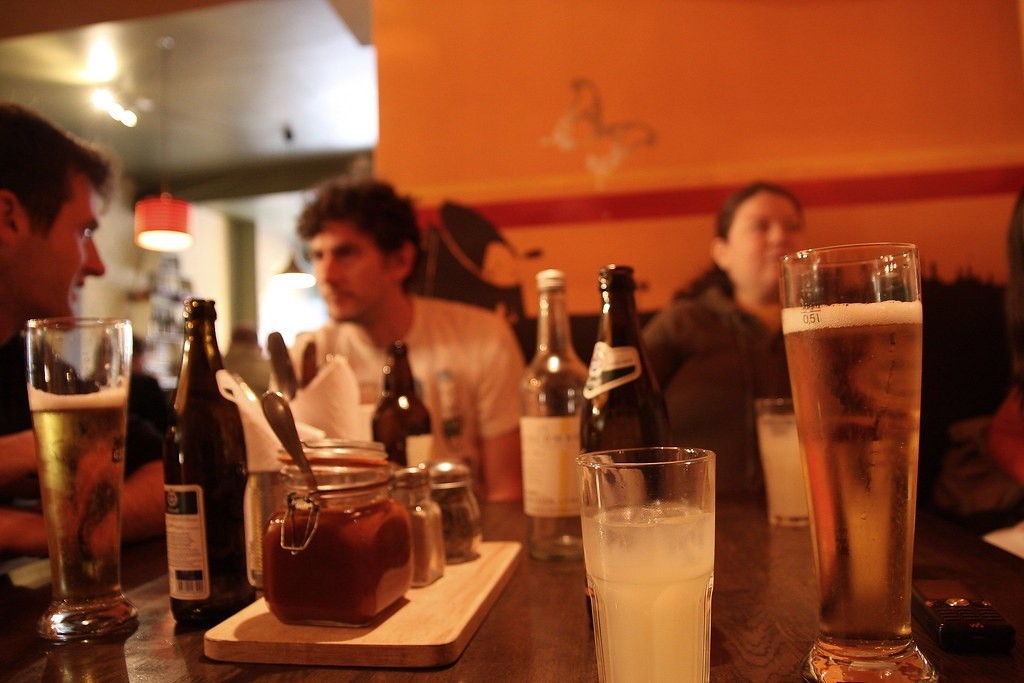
[245,372,292,589]
[431,464,480,564]
[393,467,445,586]
[580,265,677,612]
[163,299,256,627]
[373,342,433,465]
[518,270,588,562]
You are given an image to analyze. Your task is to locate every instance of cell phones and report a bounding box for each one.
[910,563,1015,653]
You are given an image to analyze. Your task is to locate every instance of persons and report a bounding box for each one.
[89,325,271,483]
[268,179,533,502]
[635,181,824,492]
[0,102,175,563]
[918,396,1024,560]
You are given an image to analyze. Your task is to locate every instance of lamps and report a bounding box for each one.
[135,49,194,254]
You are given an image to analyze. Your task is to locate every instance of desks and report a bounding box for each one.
[0,493,1024,682]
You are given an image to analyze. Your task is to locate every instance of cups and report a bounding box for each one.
[575,447,716,683]
[27,320,138,644]
[263,438,411,629]
[756,400,809,527]
[779,241,939,683]
